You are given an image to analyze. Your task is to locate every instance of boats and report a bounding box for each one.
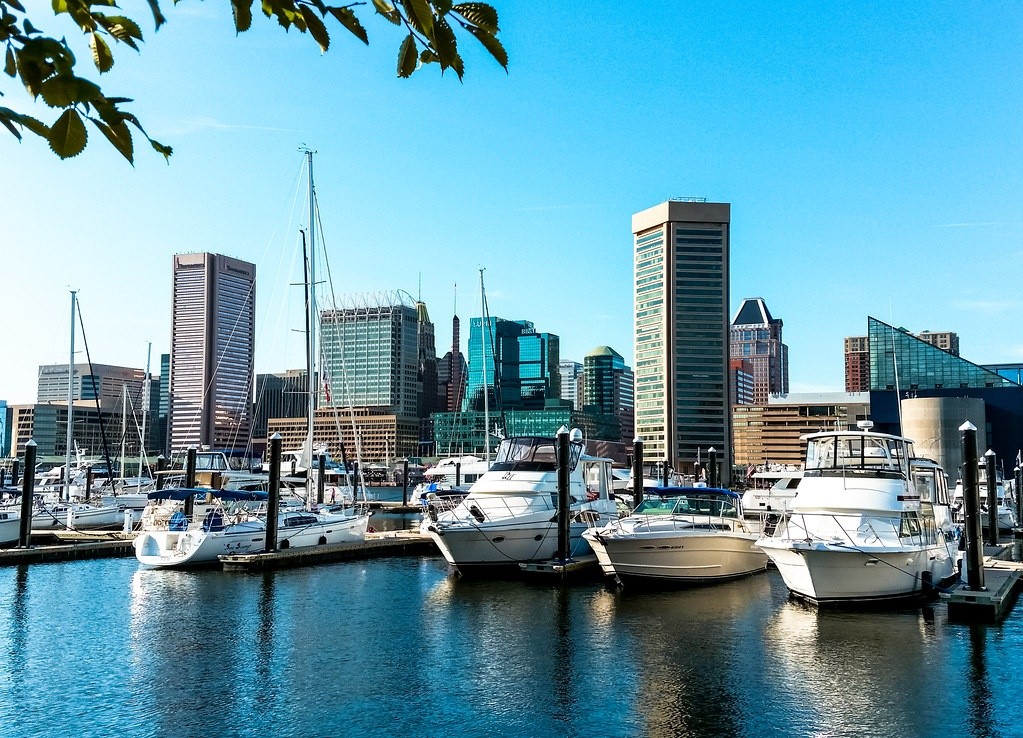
[615,443,1017,539]
[177,440,489,503]
[759,420,957,607]
[427,434,619,573]
[0,285,237,545]
[579,485,770,582]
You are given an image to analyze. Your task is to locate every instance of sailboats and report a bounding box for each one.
[132,147,376,571]
